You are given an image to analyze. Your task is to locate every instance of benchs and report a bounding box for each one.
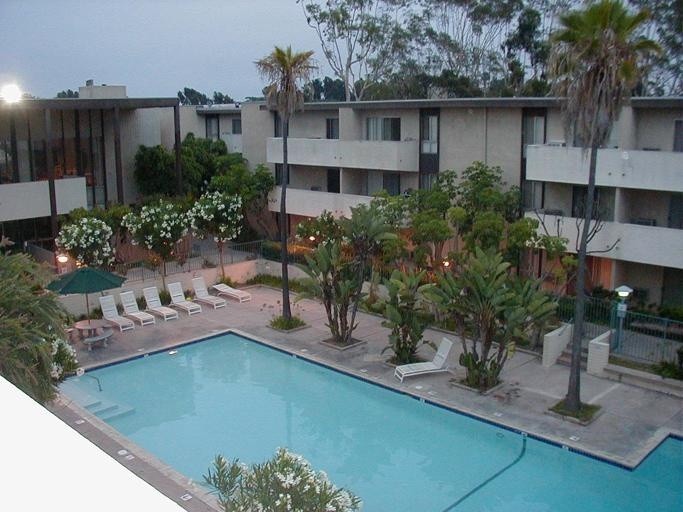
[62,328,113,352]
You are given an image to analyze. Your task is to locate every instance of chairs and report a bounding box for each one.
[393,335,458,384]
[97,275,253,334]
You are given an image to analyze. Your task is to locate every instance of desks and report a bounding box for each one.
[75,319,104,339]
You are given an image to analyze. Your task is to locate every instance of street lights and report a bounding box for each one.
[611,283,635,355]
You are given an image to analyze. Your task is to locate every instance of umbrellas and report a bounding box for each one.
[43,264,129,325]
